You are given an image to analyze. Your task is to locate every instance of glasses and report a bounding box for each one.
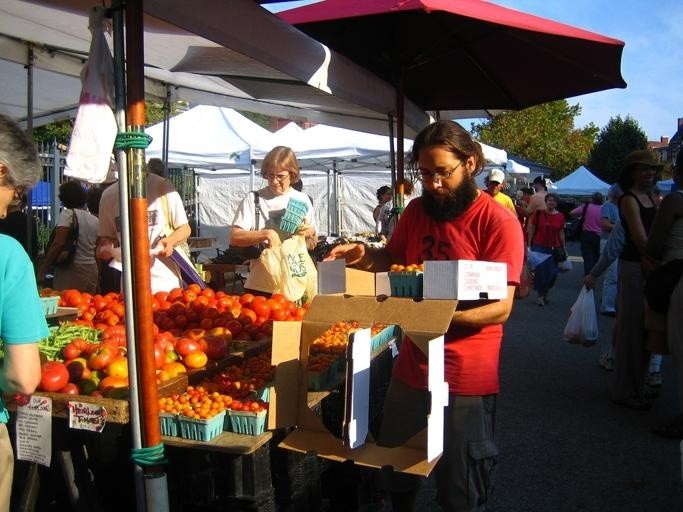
[265,173,289,181]
[6,178,29,211]
[414,160,462,181]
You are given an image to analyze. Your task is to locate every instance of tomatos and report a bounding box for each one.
[37,283,387,420]
[390,263,424,272]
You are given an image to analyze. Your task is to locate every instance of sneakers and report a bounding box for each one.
[647,372,662,386]
[536,295,551,306]
[599,353,615,369]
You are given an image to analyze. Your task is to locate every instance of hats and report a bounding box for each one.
[618,150,665,192]
[489,169,505,184]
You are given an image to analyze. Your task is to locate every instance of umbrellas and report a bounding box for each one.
[251,0,628,221]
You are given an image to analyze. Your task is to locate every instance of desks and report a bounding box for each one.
[6,338,270,512]
[202,263,236,289]
[160,430,286,511]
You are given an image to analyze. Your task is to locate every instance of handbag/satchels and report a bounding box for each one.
[229,240,264,260]
[152,225,206,290]
[553,248,566,263]
[44,208,79,266]
[578,217,585,232]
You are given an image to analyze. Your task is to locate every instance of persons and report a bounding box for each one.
[229,146,317,297]
[0,116,53,512]
[38,158,191,295]
[322,120,525,511]
[524,150,681,441]
